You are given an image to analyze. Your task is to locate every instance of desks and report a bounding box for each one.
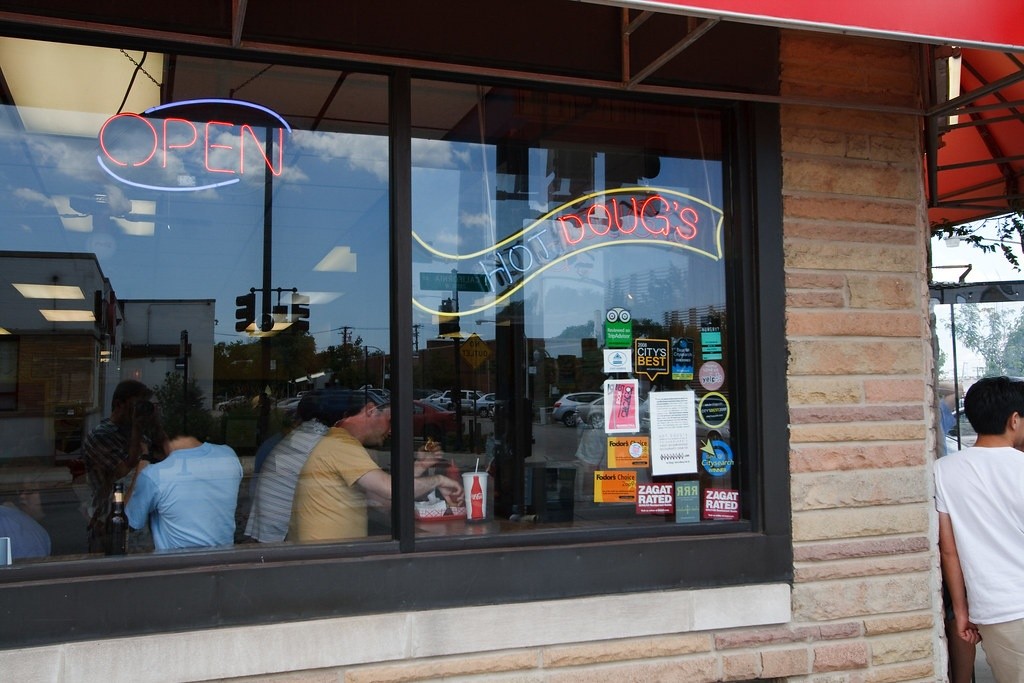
[369,508,548,536]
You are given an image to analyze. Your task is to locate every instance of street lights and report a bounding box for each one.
[475,318,533,404]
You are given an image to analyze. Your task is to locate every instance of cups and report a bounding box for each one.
[462,471,489,520]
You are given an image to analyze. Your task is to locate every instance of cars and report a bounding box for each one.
[637,398,723,440]
[333,400,466,443]
[470,393,497,418]
[580,394,646,429]
[225,388,389,428]
[422,393,442,408]
[551,391,606,427]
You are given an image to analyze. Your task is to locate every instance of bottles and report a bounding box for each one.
[471,476,483,521]
[105,483,129,558]
[447,464,462,504]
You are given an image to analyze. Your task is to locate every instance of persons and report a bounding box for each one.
[245,389,465,543]
[85,379,176,555]
[123,390,243,551]
[933,375,1024,683]
[0,464,88,558]
[256,393,271,446]
[575,367,651,517]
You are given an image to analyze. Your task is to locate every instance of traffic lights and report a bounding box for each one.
[234,291,260,333]
[437,305,464,336]
[348,343,354,356]
[292,290,315,335]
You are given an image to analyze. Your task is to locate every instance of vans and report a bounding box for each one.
[439,390,484,413]
